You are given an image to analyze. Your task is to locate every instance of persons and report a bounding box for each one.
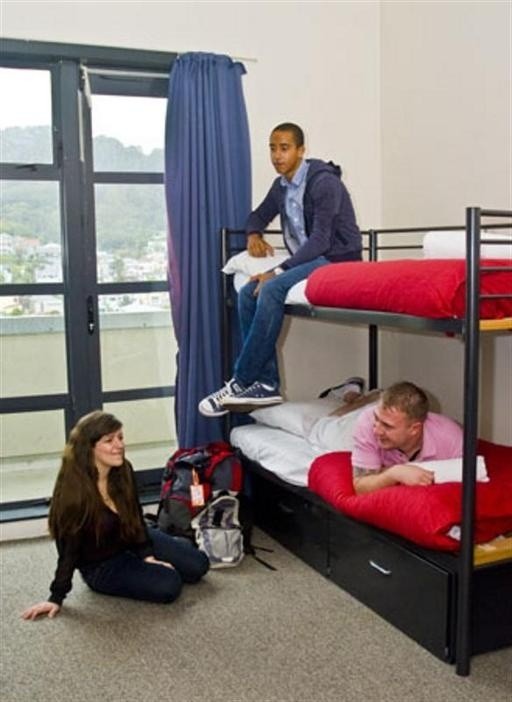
[196,122,363,417]
[317,377,463,494]
[18,409,211,621]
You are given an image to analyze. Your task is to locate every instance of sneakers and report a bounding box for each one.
[319,377,363,398]
[198,378,283,418]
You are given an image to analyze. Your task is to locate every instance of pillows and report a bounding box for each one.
[420,229,512,258]
[221,246,292,278]
[248,398,345,438]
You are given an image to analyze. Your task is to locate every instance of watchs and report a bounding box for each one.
[274,268,283,276]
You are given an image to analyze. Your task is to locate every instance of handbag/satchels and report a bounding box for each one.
[156,442,254,569]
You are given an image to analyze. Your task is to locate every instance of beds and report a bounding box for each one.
[217,206,512,677]
